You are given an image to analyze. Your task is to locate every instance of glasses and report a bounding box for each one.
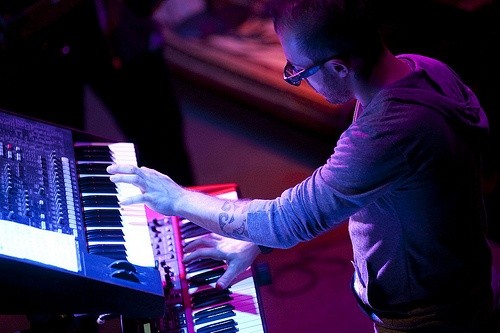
[282,51,341,87]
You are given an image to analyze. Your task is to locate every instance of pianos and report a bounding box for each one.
[132,182,266,333]
[1,107,164,319]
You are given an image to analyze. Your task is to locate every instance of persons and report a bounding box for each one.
[106,0,499,333]
[145,0,279,54]
